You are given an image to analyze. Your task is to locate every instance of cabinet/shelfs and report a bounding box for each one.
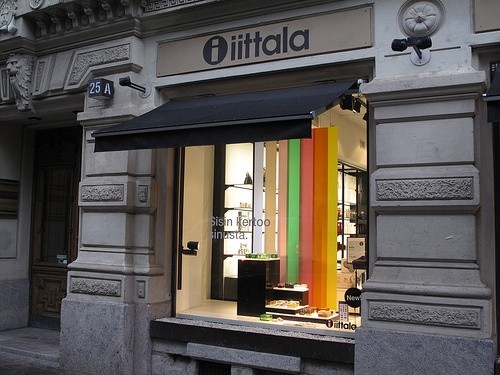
[210,143,265,300]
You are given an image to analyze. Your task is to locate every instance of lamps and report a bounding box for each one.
[390,37,432,61]
[339,94,363,113]
[117,76,152,98]
